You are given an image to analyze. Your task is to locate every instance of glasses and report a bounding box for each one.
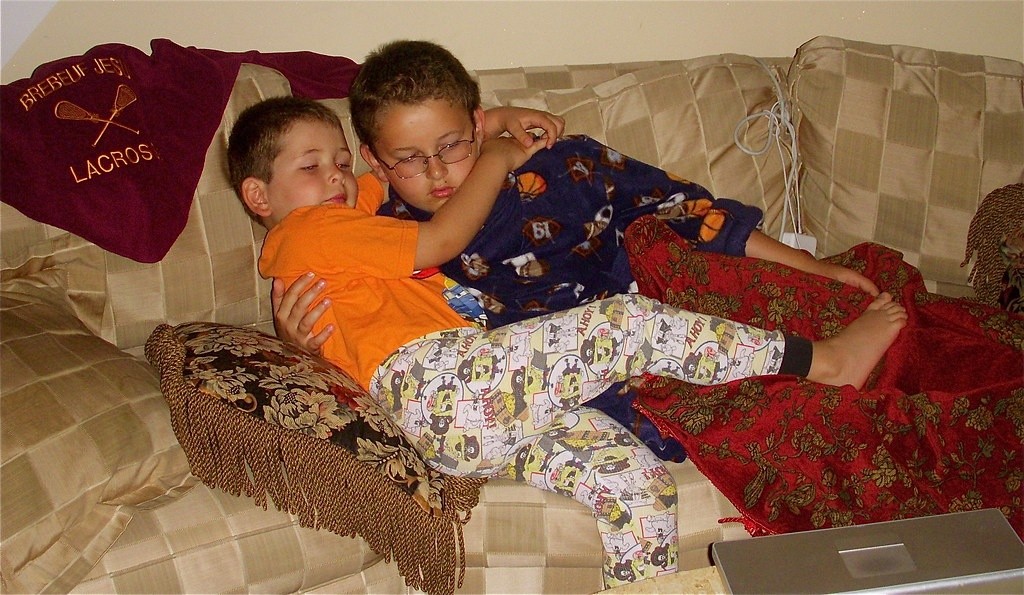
[369,123,476,180]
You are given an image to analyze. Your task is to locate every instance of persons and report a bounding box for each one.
[226,96,908,589]
[271,41,880,465]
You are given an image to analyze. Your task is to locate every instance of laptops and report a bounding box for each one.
[711,508,1024,595]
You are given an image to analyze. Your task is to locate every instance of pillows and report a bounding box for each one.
[146,319,488,595]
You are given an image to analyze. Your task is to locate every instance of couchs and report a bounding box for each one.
[0,35,1024,595]
